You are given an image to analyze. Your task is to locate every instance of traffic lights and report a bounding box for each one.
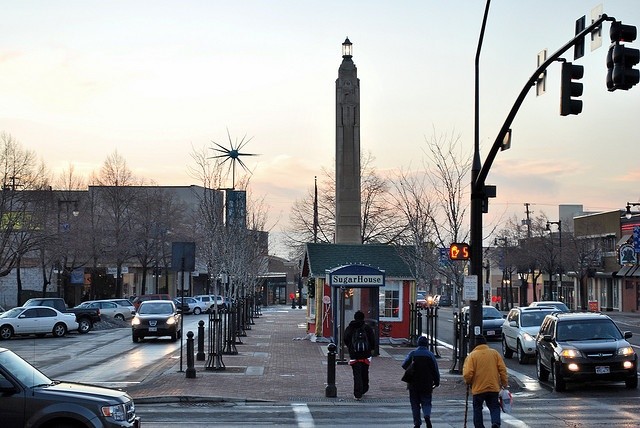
[560,61,583,117]
[449,242,471,259]
[605,21,640,92]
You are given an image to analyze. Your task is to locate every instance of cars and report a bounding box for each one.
[417,290,452,307]
[192,294,214,311]
[103,298,137,316]
[171,297,190,315]
[175,296,209,315]
[208,294,224,306]
[72,299,134,324]
[459,305,508,342]
[0,306,80,341]
[527,300,571,314]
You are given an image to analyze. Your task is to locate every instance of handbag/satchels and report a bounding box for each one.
[499,386,512,414]
[401,355,417,383]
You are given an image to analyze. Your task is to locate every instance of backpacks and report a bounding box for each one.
[348,323,371,359]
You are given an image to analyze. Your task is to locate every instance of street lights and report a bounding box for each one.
[493,236,511,312]
[151,217,172,295]
[543,219,567,304]
[51,197,80,298]
[625,200,640,220]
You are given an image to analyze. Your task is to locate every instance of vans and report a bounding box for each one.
[131,292,172,311]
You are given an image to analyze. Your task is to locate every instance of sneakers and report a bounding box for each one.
[424,417,432,428]
[492,422,500,428]
[414,424,420,428]
[354,395,363,401]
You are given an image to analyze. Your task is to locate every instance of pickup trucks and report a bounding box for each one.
[131,298,183,343]
[21,297,102,335]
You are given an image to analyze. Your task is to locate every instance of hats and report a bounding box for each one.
[476,335,486,345]
[353,310,365,321]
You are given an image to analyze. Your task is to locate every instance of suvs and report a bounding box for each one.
[533,309,639,392]
[500,305,564,366]
[0,346,142,428]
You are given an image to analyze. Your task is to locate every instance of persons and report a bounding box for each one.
[462,333,509,428]
[342,310,376,401]
[289,291,295,300]
[401,335,441,428]
[295,290,300,300]
